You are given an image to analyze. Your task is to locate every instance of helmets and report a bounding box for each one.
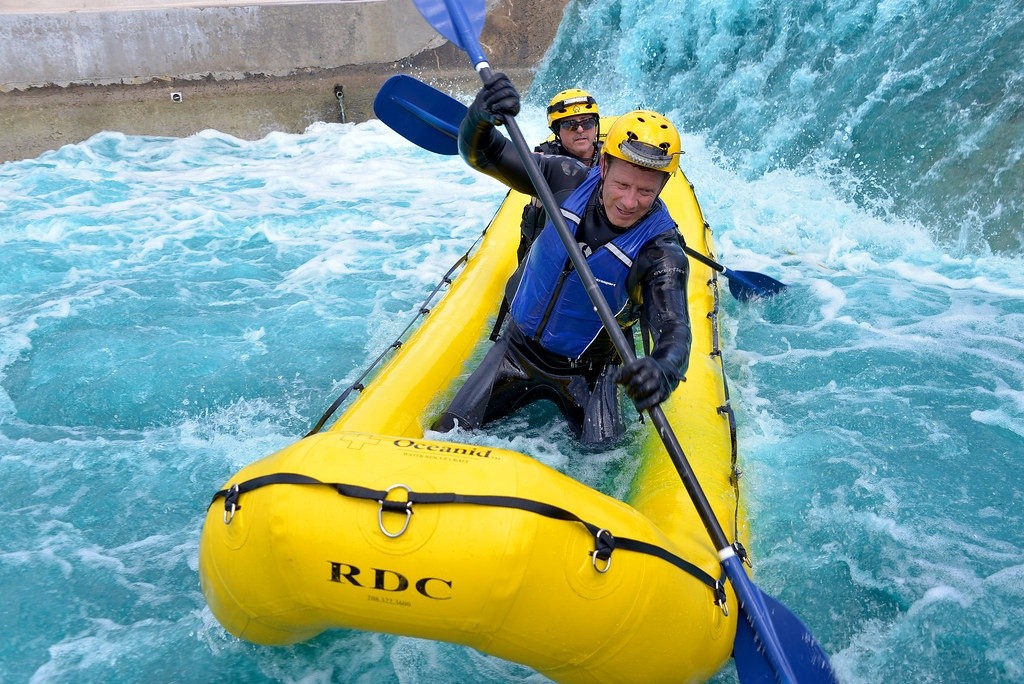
[547,89,600,135]
[600,110,685,177]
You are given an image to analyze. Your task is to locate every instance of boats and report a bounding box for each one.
[200,115,751,684]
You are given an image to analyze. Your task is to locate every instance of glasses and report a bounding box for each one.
[556,117,598,131]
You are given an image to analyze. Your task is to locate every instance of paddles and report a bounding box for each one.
[373,72,788,304]
[411,1,837,684]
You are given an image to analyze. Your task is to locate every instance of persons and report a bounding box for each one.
[534,87,604,168]
[432,71,693,443]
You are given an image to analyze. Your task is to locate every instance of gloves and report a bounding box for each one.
[620,352,686,412]
[467,73,520,133]
